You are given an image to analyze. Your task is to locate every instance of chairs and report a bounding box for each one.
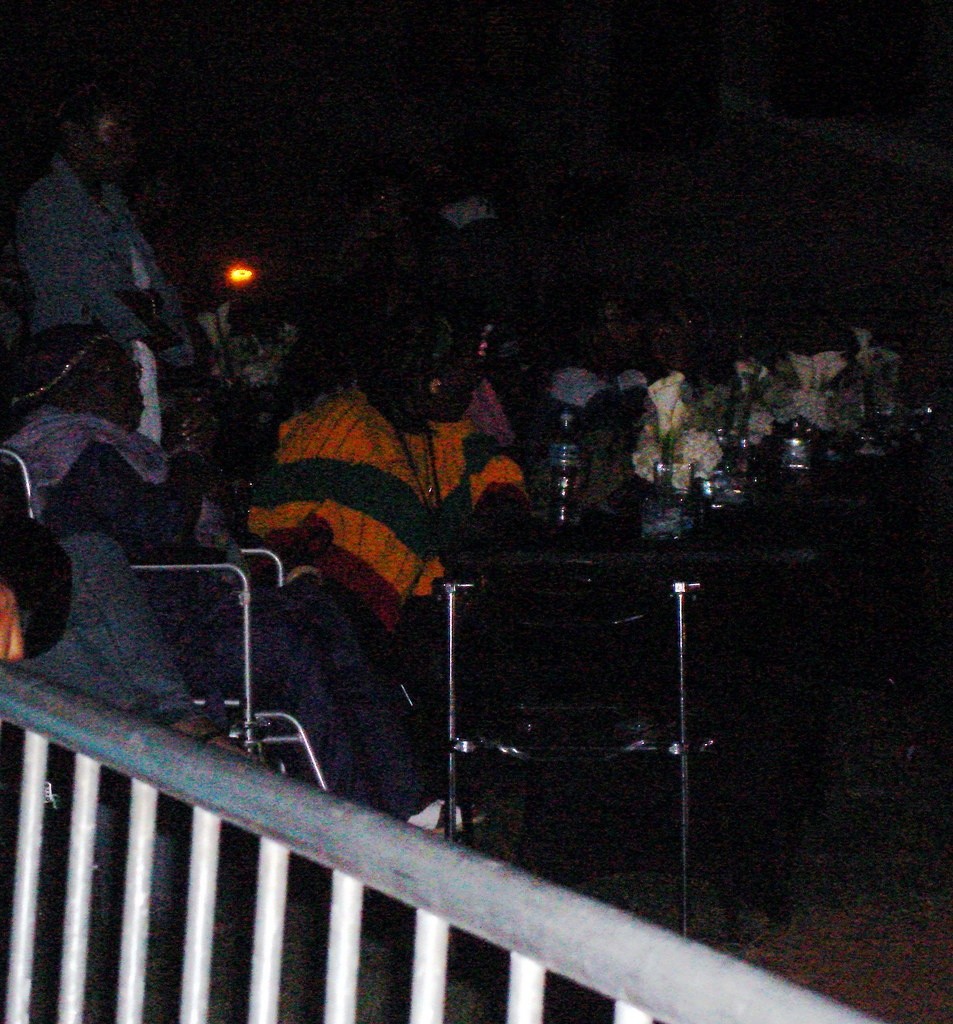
[438,556,712,936]
[0,445,332,796]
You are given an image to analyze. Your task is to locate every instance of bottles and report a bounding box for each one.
[536,404,589,531]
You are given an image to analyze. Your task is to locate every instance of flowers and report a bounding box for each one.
[629,324,904,489]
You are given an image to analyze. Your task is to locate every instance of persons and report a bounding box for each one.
[0,328,463,841]
[146,308,952,557]
[14,82,213,419]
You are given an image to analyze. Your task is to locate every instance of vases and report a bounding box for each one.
[704,448,768,508]
[638,462,688,541]
[776,422,816,470]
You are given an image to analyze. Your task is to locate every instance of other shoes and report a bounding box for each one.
[432,800,487,837]
